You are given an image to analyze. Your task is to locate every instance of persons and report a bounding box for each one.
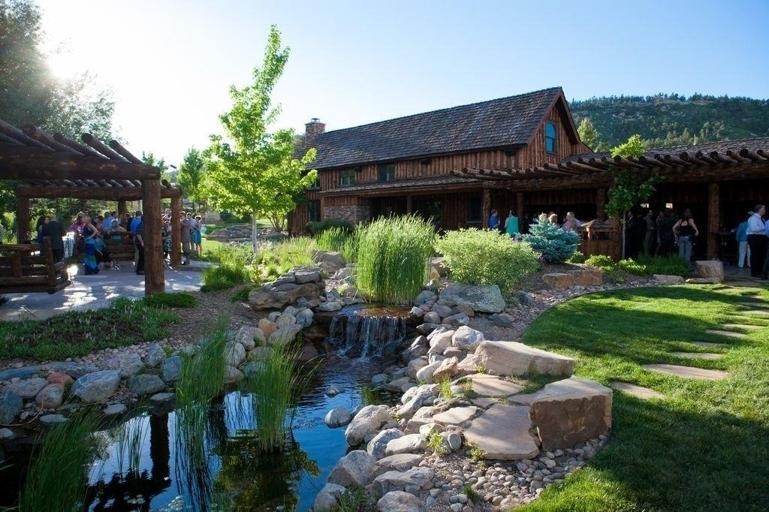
[35,208,202,275]
[489,204,769,280]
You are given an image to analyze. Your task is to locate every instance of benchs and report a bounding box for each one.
[0,237,70,294]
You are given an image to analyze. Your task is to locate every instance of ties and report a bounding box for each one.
[760,216,768,229]
